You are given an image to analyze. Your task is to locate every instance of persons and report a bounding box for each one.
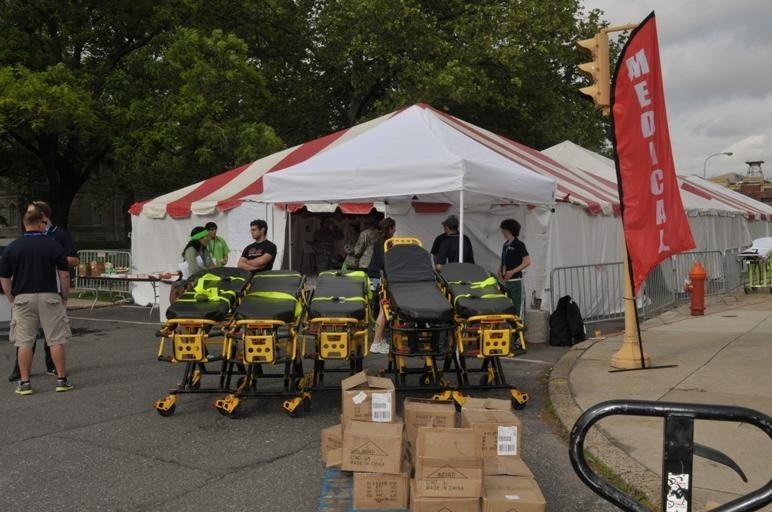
[0,207,74,394]
[435,220,475,270]
[180,226,215,360]
[237,221,277,273]
[367,218,396,353]
[8,200,81,382]
[204,222,230,268]
[500,219,531,348]
[430,215,460,269]
[290,212,385,277]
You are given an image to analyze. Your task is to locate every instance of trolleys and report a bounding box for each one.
[152,266,253,418]
[215,269,307,416]
[739,236,772,294]
[438,263,529,413]
[376,238,465,400]
[296,268,373,413]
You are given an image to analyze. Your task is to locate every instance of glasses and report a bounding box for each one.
[42,221,47,225]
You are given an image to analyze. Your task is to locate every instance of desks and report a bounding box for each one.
[74,275,162,318]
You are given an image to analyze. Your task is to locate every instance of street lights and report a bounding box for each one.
[704,151,734,179]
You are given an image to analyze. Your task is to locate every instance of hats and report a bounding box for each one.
[442,217,458,230]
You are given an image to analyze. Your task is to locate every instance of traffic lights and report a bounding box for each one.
[574,35,598,107]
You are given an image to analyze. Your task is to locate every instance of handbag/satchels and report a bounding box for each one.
[178,256,205,280]
[551,295,586,345]
[346,245,374,268]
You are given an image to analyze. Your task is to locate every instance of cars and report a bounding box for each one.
[1,238,67,338]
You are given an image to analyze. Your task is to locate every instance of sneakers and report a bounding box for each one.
[512,343,527,354]
[370,340,390,354]
[8,371,33,395]
[47,368,74,392]
[207,354,216,360]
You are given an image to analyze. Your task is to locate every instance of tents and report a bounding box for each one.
[127,102,652,321]
[539,139,772,295]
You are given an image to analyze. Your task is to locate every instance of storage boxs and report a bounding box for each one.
[320,372,546,512]
